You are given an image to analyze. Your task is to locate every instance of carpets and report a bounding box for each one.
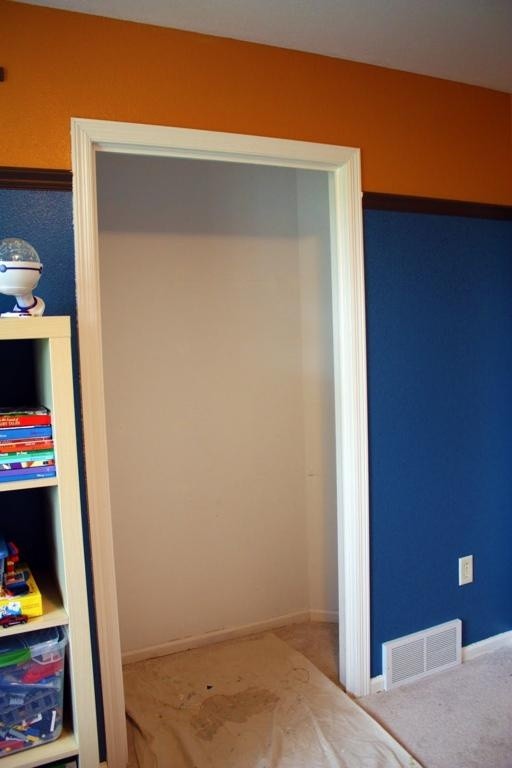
[123,630,427,767]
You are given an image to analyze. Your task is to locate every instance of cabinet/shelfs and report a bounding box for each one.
[0,316,100,766]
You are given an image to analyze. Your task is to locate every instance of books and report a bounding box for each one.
[0,405,56,482]
